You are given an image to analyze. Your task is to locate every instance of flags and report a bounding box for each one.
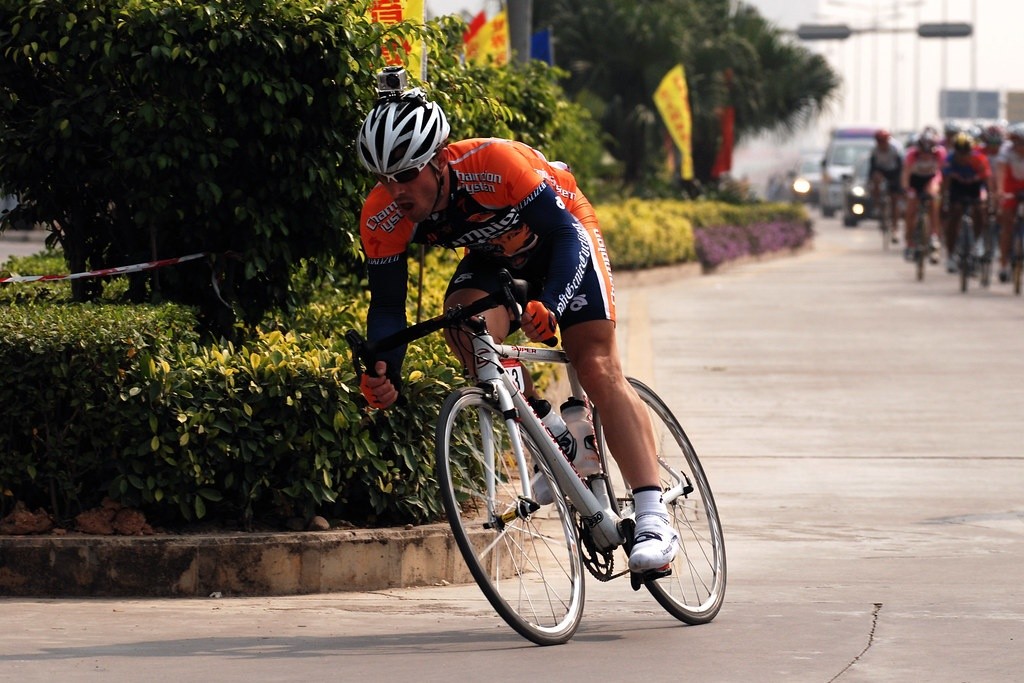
[463,13,508,70]
[357,0,427,83]
[714,66,735,174]
[653,64,695,180]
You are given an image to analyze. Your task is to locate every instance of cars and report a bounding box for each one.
[762,148,825,205]
[840,153,907,227]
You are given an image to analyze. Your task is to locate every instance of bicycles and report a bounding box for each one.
[342,267,728,647]
[870,174,1024,293]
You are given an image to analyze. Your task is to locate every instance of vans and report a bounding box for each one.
[819,125,907,218]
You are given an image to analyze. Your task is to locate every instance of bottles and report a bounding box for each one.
[560,397,601,477]
[526,395,576,461]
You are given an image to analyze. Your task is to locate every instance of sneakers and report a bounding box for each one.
[531,432,578,506]
[628,517,678,573]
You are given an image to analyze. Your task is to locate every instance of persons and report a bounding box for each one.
[867,123,1024,281]
[362,66,680,571]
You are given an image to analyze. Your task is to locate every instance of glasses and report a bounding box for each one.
[375,141,450,184]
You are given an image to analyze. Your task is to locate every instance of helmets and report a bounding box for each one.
[876,121,1023,152]
[356,87,449,173]
